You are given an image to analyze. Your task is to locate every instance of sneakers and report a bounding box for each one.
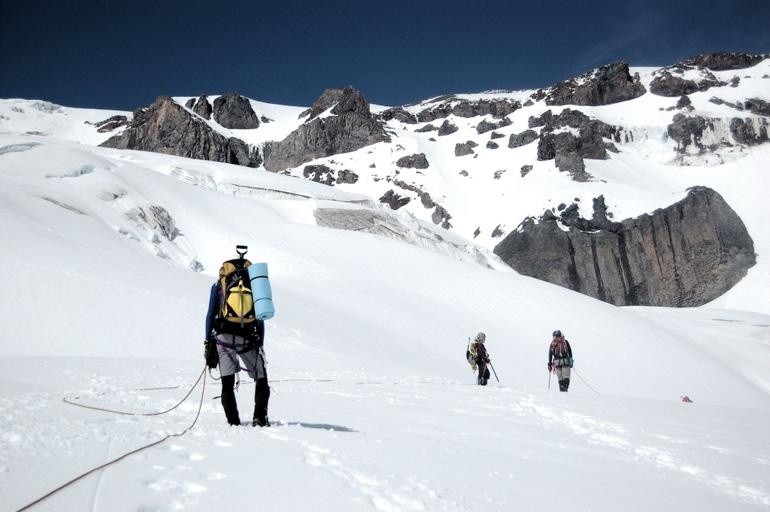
[252,409,270,427]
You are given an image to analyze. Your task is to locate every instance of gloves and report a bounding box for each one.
[204,339,219,369]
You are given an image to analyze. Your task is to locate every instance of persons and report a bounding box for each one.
[204,267,270,427]
[548,330,574,392]
[474,333,490,386]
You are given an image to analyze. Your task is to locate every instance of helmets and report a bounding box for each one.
[552,330,562,338]
[475,332,486,343]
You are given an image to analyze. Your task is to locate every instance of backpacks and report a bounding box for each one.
[217,259,253,326]
[467,343,477,370]
[552,336,568,359]
